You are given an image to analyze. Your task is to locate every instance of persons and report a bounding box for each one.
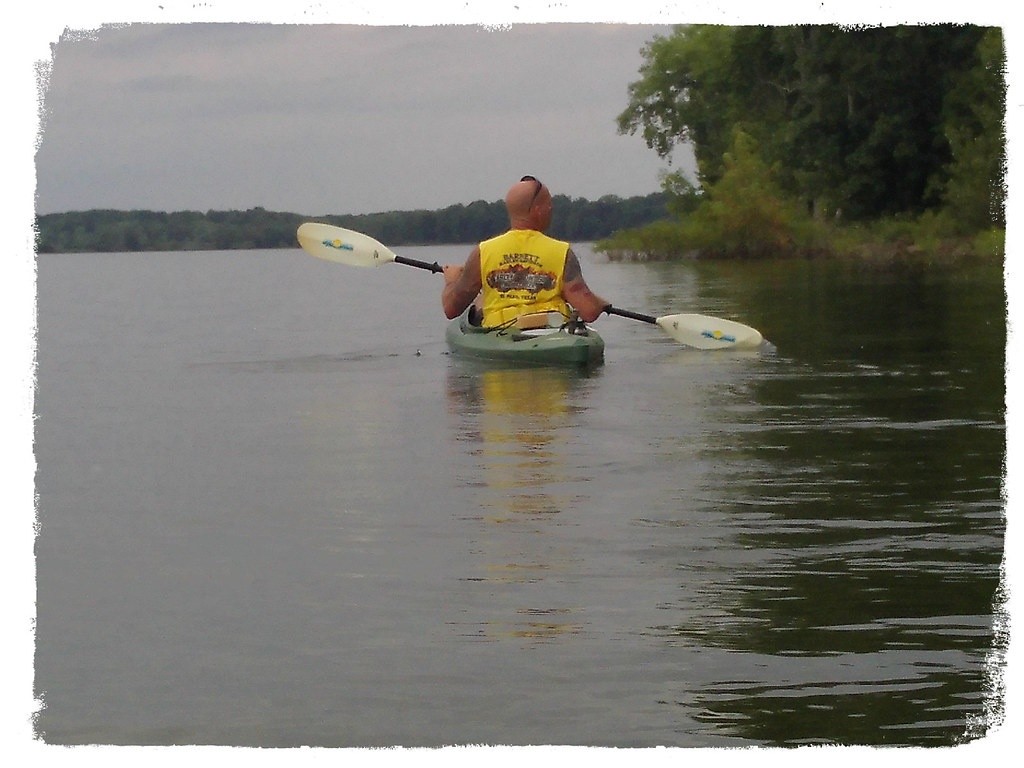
[442,175,608,328]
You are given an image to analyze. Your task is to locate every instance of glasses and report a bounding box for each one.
[521,173,542,215]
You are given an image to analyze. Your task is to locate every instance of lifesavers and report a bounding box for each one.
[296,221,763,348]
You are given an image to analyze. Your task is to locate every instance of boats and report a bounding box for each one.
[445,302,605,366]
